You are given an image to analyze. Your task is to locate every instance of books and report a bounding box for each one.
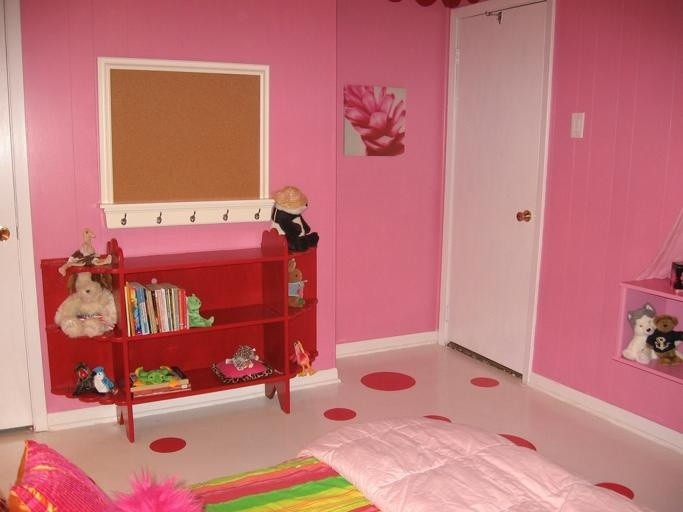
[122,280,190,337]
[129,367,191,398]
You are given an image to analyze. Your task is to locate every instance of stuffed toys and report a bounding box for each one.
[621,304,660,365]
[647,315,682,366]
[287,257,308,309]
[272,186,319,251]
[71,361,101,396]
[57,228,114,277]
[188,292,214,328]
[134,365,174,385]
[53,271,118,339]
[288,338,318,378]
[89,365,114,395]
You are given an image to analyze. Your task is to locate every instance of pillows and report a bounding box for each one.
[7,438,124,512]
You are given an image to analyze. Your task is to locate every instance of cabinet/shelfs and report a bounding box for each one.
[610,278,682,385]
[40,227,318,442]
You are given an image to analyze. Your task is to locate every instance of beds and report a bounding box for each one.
[119,417,643,512]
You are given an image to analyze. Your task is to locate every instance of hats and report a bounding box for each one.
[271,186,308,210]
